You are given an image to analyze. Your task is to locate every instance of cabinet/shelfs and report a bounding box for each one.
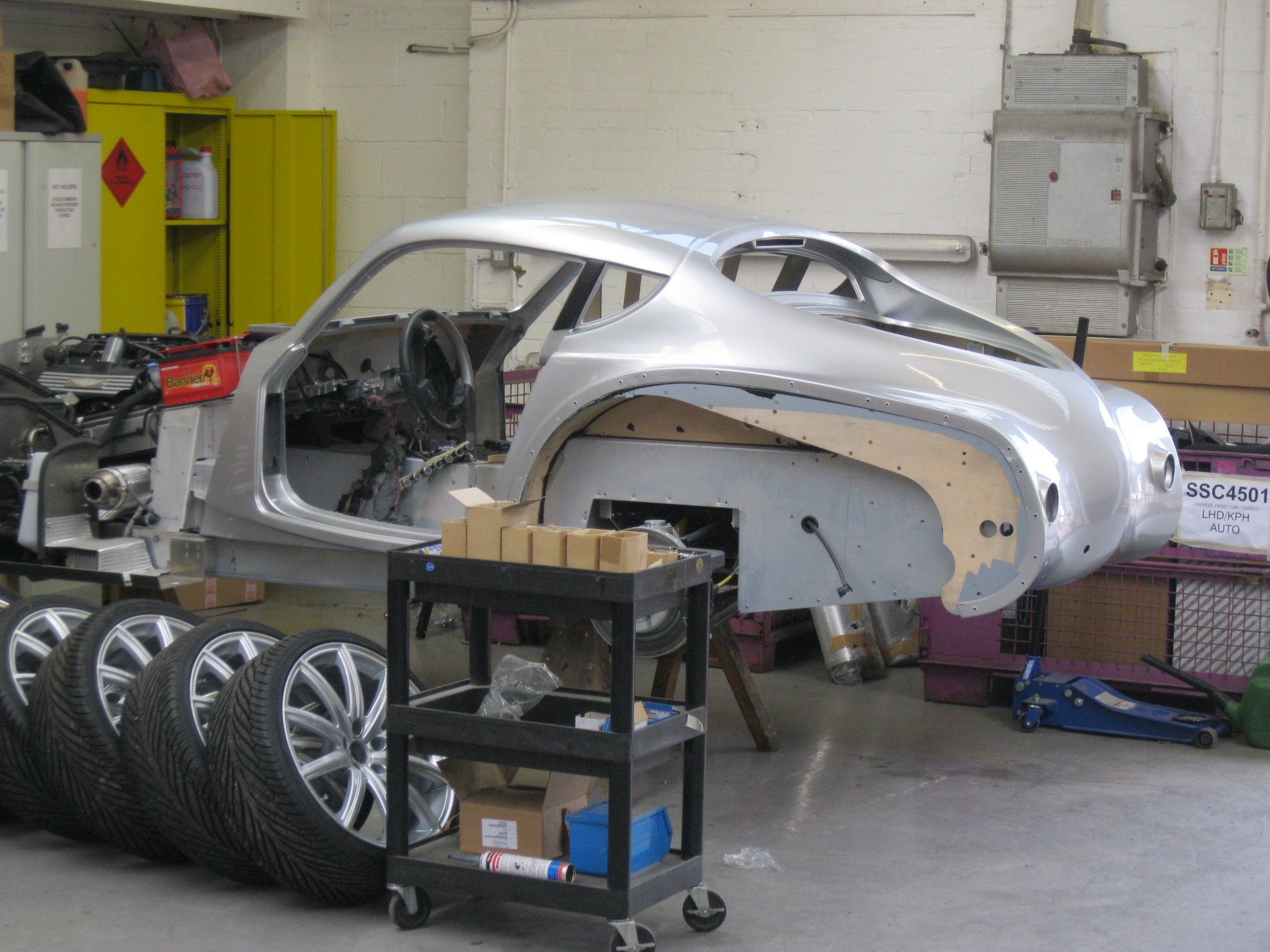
[388,537,729,952]
[68,86,348,341]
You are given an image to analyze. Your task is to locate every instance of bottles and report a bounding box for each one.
[54,59,88,134]
[474,851,577,883]
[164,141,184,220]
[181,146,218,220]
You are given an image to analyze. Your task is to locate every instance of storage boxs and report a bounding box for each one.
[98,567,270,612]
